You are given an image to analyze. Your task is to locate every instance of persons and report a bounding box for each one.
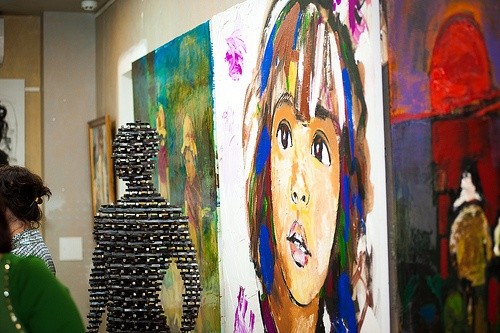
[86,120,203,333]
[0,165,57,280]
[0,197,86,333]
[0,148,11,166]
